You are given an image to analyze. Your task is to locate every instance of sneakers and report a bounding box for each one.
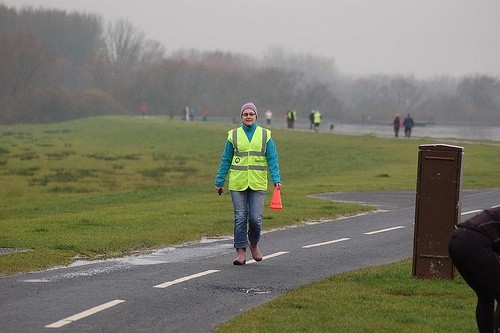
[233,248,246,265]
[249,242,262,261]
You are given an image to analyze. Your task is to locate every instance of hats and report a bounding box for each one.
[240,101,258,121]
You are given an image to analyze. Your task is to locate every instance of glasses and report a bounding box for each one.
[242,112,256,116]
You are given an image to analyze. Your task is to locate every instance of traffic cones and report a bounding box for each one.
[269,186,284,209]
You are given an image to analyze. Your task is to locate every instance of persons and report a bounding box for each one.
[141,106,146,116]
[170,106,209,121]
[215,103,282,265]
[264,107,322,131]
[393,113,414,138]
[449,206,500,333]
